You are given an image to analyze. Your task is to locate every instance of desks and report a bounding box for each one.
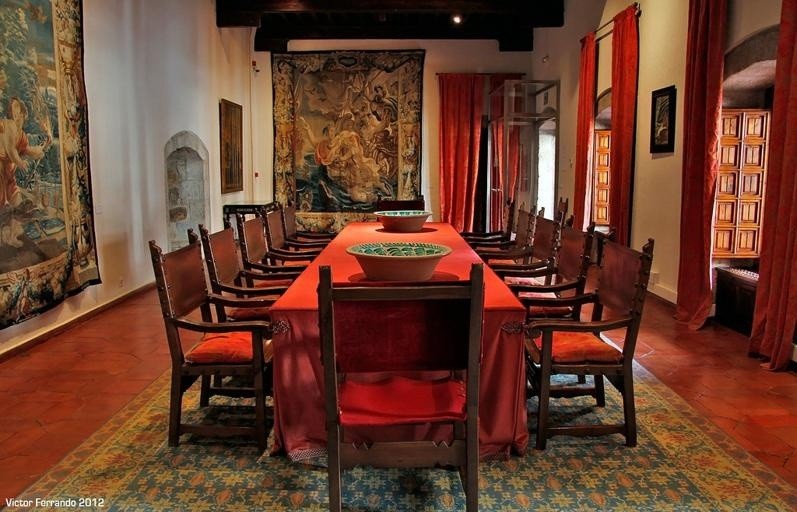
[268,223,530,460]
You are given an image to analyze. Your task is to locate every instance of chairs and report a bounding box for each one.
[318,264,483,511]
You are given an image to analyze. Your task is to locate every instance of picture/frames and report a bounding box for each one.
[650,85,677,152]
[219,98,243,194]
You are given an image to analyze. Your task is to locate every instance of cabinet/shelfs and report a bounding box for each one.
[715,266,760,332]
[712,109,771,260]
[590,130,612,225]
[489,78,561,217]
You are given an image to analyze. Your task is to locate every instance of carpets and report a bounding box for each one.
[1,333,797,512]
